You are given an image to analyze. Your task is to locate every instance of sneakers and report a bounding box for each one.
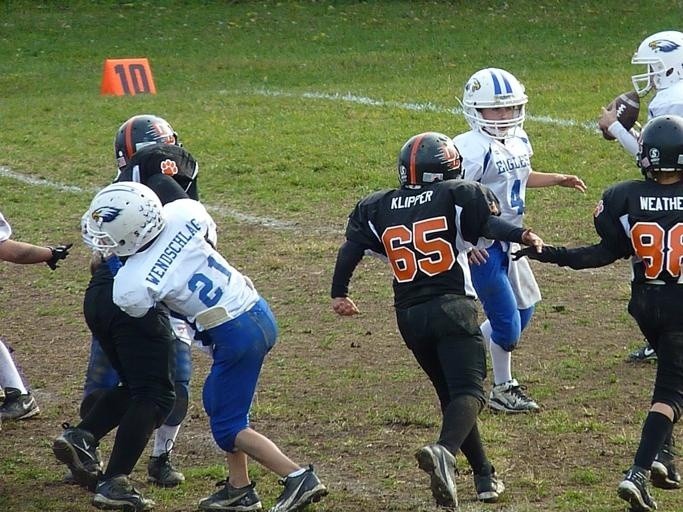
[0,387,40,421]
[487,378,539,415]
[198,482,262,512]
[415,445,457,507]
[267,470,328,512]
[627,344,657,363]
[52,427,185,512]
[616,466,656,512]
[473,465,504,502]
[650,452,679,490]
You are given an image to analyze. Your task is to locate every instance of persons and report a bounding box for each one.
[331,131,553,507]
[79,113,191,487]
[51,144,199,511]
[445,66,588,413]
[0,210,73,423]
[597,29,683,158]
[80,181,327,512]
[511,113,683,511]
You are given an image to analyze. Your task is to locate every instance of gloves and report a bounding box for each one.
[43,244,73,270]
[512,245,561,264]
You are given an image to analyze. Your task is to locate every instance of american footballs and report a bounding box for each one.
[602,92,640,140]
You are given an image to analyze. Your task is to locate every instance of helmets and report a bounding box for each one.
[115,115,177,172]
[399,131,460,187]
[462,68,527,140]
[635,114,682,180]
[631,30,683,97]
[81,181,163,258]
